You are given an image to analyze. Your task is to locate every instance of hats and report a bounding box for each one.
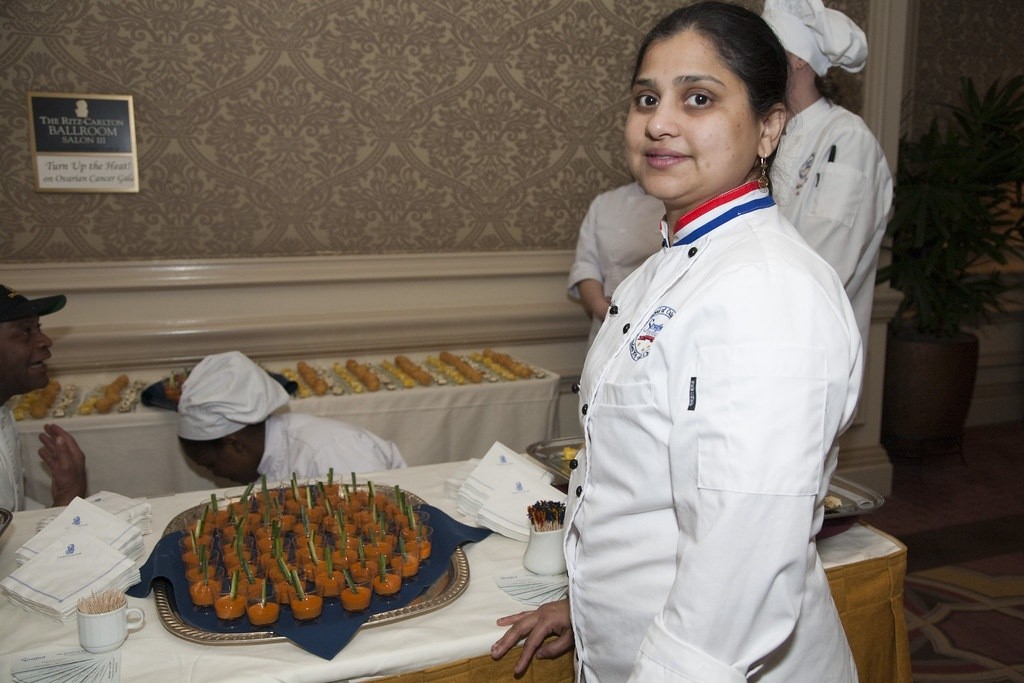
[177,351,289,442]
[760,0,869,77]
[0,284,66,324]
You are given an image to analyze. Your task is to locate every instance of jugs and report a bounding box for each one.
[522,525,567,575]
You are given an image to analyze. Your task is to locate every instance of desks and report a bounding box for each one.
[13,347,562,508]
[0,454,914,683]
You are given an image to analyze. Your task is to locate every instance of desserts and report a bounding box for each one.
[8,373,145,421]
[179,466,431,626]
[284,349,532,398]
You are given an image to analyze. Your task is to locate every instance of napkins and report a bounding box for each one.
[0,490,155,633]
[443,440,566,542]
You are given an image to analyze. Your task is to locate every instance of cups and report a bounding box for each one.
[76,596,145,654]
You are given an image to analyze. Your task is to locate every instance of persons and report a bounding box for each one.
[566,182,666,359]
[760,0,893,367]
[491,0,864,683]
[0,284,87,512]
[177,350,408,486]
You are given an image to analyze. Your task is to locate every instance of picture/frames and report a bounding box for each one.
[27,90,139,193]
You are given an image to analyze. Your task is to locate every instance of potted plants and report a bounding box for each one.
[875,75,1024,455]
